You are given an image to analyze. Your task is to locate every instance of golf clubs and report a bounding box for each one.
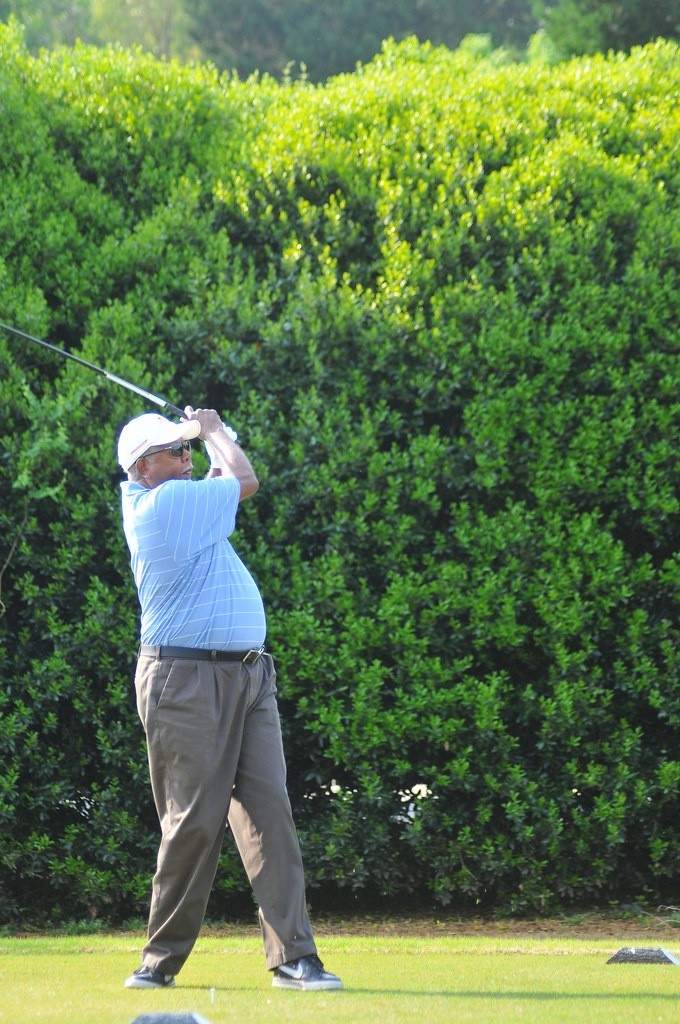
[0,321,246,447]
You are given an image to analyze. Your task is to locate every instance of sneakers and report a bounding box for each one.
[125,965,175,989]
[272,955,343,991]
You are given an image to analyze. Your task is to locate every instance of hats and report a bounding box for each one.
[117,413,201,472]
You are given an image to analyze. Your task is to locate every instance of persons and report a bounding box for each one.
[116,405,343,991]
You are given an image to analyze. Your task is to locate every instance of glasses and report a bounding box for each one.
[134,441,190,464]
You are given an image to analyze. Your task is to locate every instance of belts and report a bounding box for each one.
[141,644,266,668]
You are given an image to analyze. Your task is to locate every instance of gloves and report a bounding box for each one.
[204,422,238,468]
